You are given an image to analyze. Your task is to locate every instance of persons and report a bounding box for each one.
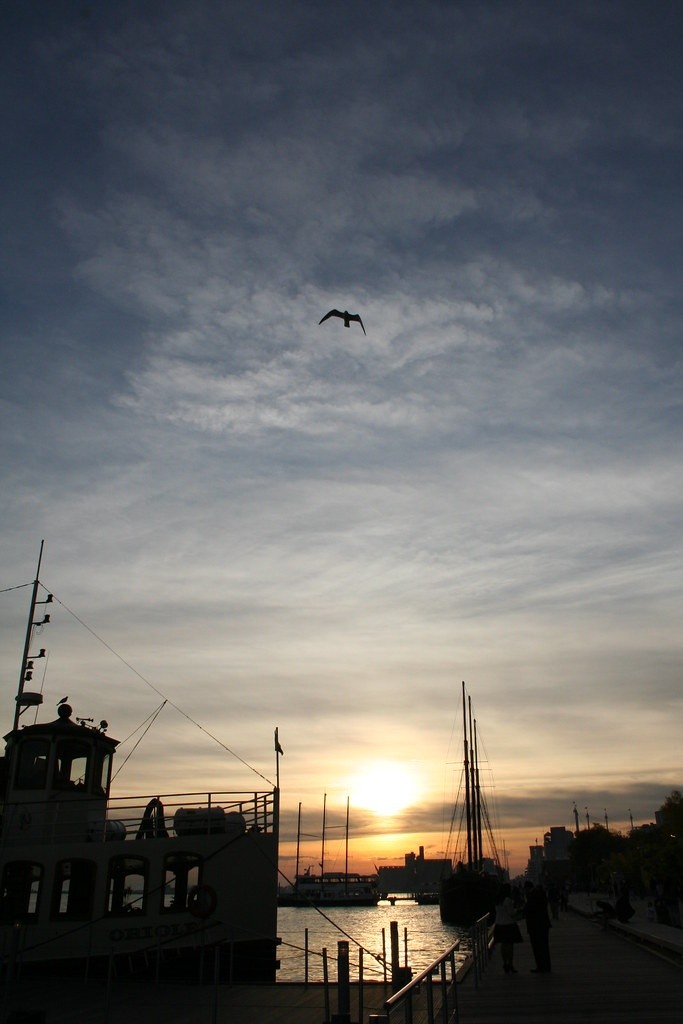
[512,878,568,921]
[517,881,553,974]
[495,883,523,973]
[594,874,680,931]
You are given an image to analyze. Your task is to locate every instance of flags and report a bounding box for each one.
[275,729,283,755]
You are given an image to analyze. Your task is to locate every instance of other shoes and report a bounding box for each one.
[601,926,608,932]
[530,966,550,973]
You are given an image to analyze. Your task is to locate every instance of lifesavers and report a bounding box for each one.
[188,885,218,919]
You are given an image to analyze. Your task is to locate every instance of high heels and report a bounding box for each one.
[502,962,518,973]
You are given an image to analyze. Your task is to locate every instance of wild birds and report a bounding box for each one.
[318,308,367,336]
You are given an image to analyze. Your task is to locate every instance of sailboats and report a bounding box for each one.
[0,537,284,1024]
[437,678,513,923]
[275,791,390,905]
